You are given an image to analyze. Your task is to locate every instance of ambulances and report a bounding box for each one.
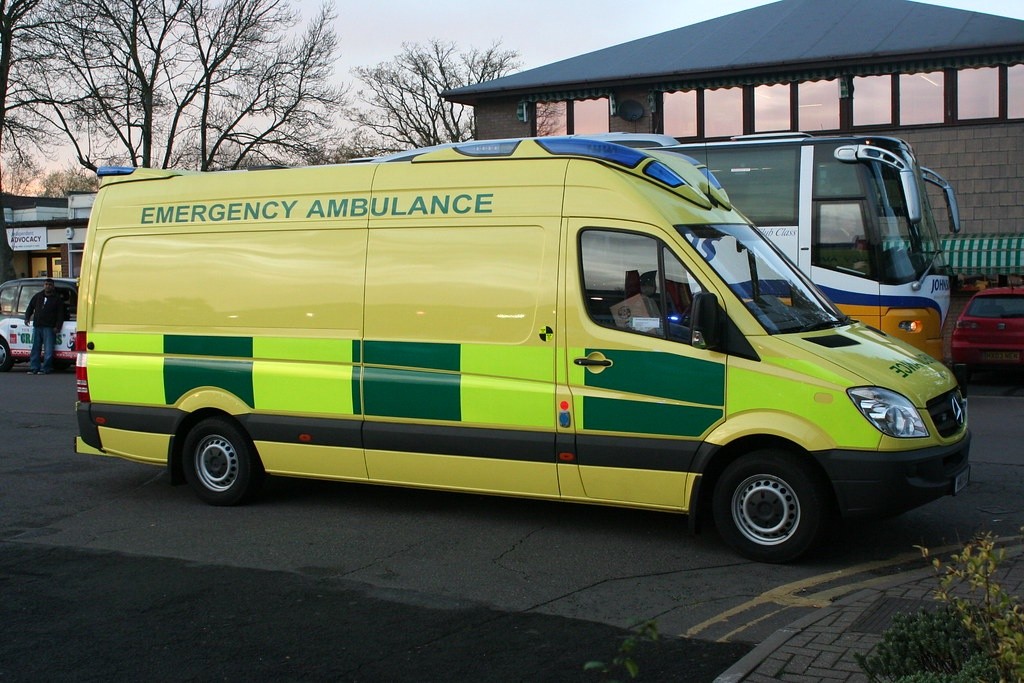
[71,133,976,560]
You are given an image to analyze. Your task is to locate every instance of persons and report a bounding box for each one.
[24,279,66,375]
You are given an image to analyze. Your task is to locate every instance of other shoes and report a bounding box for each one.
[26,368,36,375]
[37,370,47,376]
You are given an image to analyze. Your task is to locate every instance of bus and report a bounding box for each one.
[654,138,954,371]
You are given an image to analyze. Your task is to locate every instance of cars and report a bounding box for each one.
[954,288,1024,386]
[0,279,79,372]
